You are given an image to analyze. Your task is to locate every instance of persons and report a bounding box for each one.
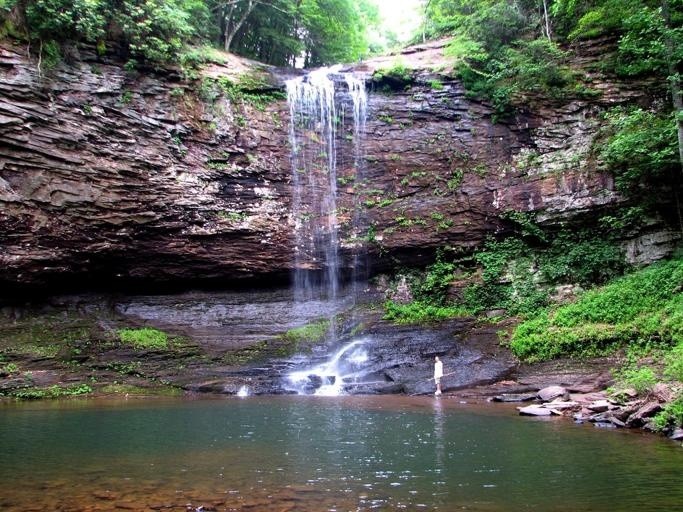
[433,356,444,395]
